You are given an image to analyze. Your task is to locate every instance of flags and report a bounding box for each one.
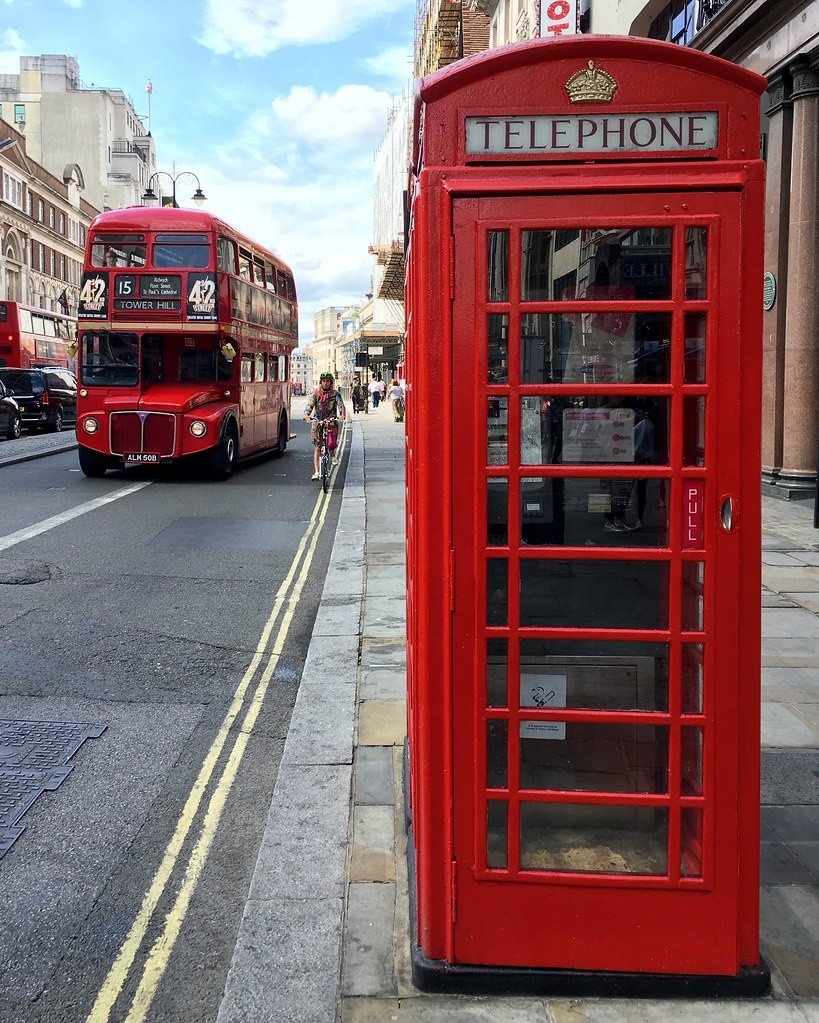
[57,291,69,309]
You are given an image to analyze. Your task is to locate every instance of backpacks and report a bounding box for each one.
[353,381,359,393]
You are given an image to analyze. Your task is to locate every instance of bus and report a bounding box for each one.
[293,382,302,396]
[74,206,298,482]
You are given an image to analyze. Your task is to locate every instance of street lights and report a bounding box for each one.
[141,171,207,209]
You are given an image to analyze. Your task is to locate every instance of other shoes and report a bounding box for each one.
[331,457,338,466]
[311,474,319,480]
[604,517,643,534]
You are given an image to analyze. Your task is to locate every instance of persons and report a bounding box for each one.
[386,379,405,423]
[112,345,137,377]
[303,371,346,481]
[103,251,119,268]
[368,376,387,407]
[349,375,364,414]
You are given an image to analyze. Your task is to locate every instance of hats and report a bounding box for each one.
[320,371,333,379]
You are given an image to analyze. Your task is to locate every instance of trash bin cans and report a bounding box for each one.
[355,397,364,411]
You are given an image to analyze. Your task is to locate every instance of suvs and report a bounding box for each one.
[0,366,78,431]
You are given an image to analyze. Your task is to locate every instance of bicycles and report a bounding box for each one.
[303,415,344,492]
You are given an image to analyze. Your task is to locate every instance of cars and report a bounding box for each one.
[0,379,21,441]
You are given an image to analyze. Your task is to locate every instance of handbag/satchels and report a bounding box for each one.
[327,422,337,450]
